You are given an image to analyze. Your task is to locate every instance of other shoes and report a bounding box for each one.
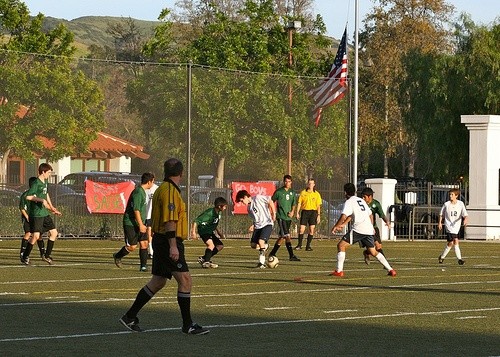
[140,266,150,272]
[295,246,302,250]
[147,254,153,259]
[363,250,370,265]
[41,253,46,261]
[306,247,312,251]
[20,251,24,257]
[265,244,269,252]
[258,263,266,269]
[458,259,466,265]
[197,257,205,265]
[203,262,219,268]
[113,253,122,268]
[438,255,444,264]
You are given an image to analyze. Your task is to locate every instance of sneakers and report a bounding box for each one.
[387,270,396,276]
[268,254,276,257]
[182,321,210,336]
[329,270,343,277]
[119,314,141,333]
[42,256,55,265]
[289,255,300,262]
[22,255,29,265]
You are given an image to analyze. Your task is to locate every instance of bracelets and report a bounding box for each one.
[318,214,320,215]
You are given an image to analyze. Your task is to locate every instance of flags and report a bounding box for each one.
[307,21,348,126]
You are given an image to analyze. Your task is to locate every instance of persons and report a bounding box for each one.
[113,172,161,272]
[330,183,397,277]
[235,190,277,270]
[268,175,301,261]
[295,178,322,251]
[361,187,392,270]
[192,197,228,268]
[120,158,210,335]
[20,163,62,265]
[438,188,468,266]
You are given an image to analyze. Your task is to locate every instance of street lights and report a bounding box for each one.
[286,18,301,190]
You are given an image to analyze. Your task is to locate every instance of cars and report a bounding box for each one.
[0,169,469,239]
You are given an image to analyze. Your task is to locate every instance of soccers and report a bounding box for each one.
[266,256,279,269]
